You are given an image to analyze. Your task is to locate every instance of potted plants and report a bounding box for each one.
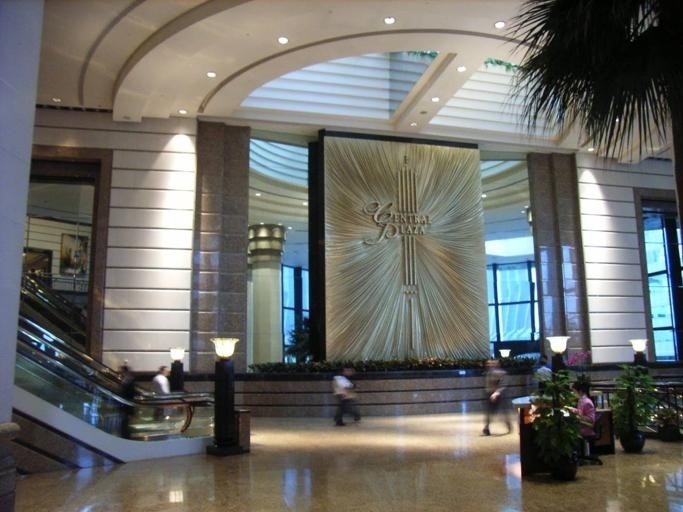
[534,366,578,478]
[614,364,657,452]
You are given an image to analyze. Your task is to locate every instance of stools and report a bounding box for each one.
[581,431,604,466]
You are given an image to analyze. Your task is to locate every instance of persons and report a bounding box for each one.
[481,359,514,435]
[330,367,360,427]
[563,380,597,437]
[532,354,553,395]
[151,365,171,419]
[118,366,135,439]
[29,268,38,276]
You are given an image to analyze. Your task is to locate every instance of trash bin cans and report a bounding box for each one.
[235,409,250,452]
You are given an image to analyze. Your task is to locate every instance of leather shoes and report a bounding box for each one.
[482,427,490,436]
[336,421,345,426]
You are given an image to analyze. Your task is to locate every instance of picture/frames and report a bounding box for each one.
[60,233,89,276]
